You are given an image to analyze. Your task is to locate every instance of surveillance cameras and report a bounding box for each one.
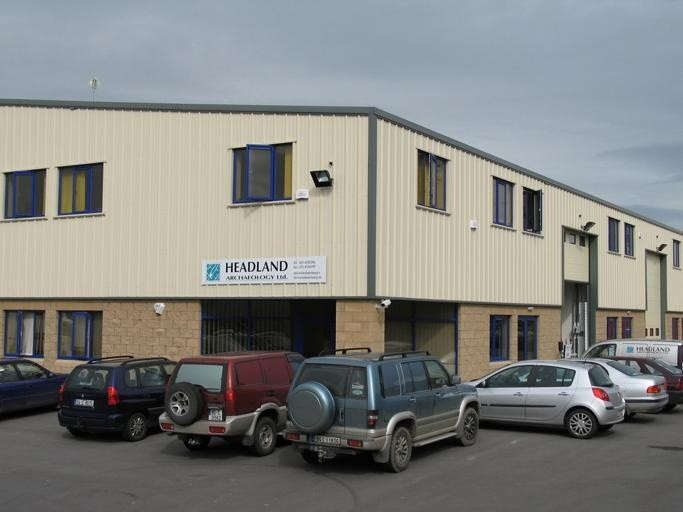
[381,299,391,308]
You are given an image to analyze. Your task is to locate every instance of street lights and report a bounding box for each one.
[90,79,98,102]
